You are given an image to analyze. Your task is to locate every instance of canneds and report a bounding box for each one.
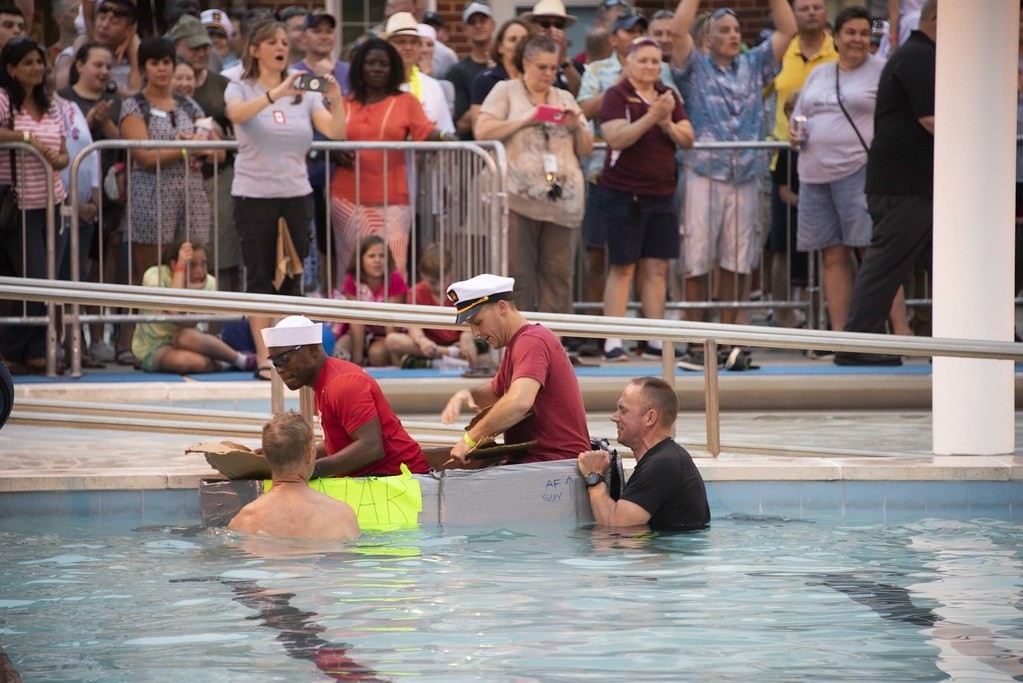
[793,115,807,142]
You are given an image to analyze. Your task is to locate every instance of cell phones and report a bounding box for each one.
[299,75,330,93]
[534,104,568,125]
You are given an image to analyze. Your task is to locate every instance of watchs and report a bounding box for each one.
[584,471,606,491]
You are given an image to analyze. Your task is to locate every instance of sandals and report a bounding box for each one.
[255,366,273,381]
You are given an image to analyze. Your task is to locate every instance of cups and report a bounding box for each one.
[439,355,470,375]
[192,120,212,141]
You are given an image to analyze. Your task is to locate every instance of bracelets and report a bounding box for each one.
[182,149,186,159]
[315,463,323,477]
[440,131,447,140]
[175,265,185,272]
[23,131,29,143]
[266,91,275,104]
[464,432,476,448]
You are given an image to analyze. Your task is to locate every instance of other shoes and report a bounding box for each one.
[230,350,257,371]
[552,316,908,368]
[399,355,433,369]
[0,338,143,375]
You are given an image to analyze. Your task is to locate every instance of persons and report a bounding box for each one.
[131,240,256,374]
[223,22,347,383]
[228,410,362,544]
[577,379,712,532]
[598,35,695,362]
[340,235,412,368]
[669,0,799,373]
[248,315,435,479]
[461,33,596,377]
[792,0,938,367]
[60,43,128,375]
[0,1,937,283]
[443,274,592,464]
[119,33,225,367]
[1,39,71,382]
[404,241,492,379]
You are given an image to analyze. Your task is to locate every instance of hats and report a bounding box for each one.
[383,11,423,42]
[261,316,323,347]
[611,13,661,56]
[201,9,234,42]
[423,11,445,28]
[170,13,214,49]
[523,0,577,29]
[461,0,494,24]
[302,7,335,31]
[447,273,515,323]
[416,23,437,46]
[98,0,136,18]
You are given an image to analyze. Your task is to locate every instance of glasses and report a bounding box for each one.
[597,0,630,15]
[711,6,739,21]
[270,345,303,367]
[531,17,566,30]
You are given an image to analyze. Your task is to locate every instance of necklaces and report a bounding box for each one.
[409,65,420,102]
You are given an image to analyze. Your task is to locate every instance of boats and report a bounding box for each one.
[196,439,623,543]
